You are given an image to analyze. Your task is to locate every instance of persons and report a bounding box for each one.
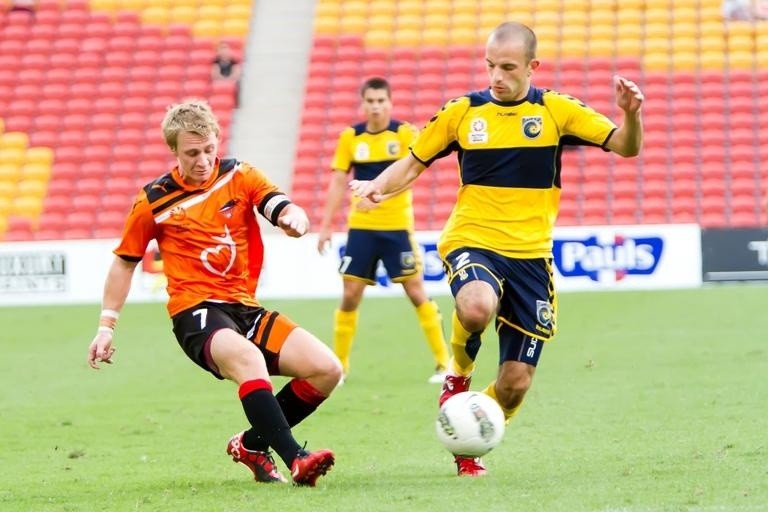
[209,44,242,83]
[86,97,344,487]
[319,77,451,387]
[349,22,646,479]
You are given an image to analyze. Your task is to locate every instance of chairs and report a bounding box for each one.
[1,0,252,241]
[287,1,768,233]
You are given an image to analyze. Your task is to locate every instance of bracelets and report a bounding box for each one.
[97,310,118,334]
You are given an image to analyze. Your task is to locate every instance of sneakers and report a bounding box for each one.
[439,355,473,406]
[452,450,486,476]
[290,449,335,486]
[227,431,287,483]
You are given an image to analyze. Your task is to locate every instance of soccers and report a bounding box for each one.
[435,391,505,456]
